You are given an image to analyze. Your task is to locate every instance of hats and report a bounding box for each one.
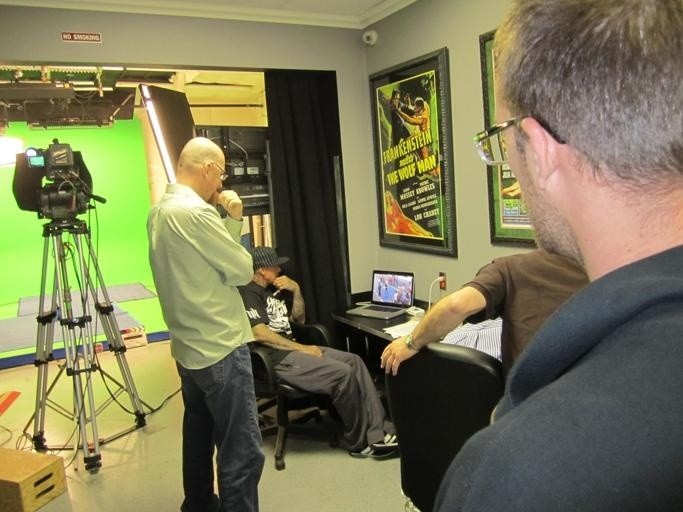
[252,246,290,270]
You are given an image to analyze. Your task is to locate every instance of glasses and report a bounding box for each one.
[213,161,229,181]
[473,113,568,166]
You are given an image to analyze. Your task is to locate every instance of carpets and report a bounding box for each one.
[18,282,158,316]
[1,301,141,354]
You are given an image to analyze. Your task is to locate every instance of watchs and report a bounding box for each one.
[405,334,421,351]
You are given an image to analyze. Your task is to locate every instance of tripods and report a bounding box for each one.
[32,219,146,471]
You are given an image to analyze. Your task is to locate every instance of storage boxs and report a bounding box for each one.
[0,448,69,511]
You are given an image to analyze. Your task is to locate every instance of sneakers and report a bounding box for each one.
[373,432,399,448]
[351,446,397,458]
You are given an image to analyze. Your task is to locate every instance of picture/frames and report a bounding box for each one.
[368,46,458,258]
[479,30,537,248]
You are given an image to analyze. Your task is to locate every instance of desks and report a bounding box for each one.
[332,302,421,421]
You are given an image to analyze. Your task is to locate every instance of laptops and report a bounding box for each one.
[346,270,415,320]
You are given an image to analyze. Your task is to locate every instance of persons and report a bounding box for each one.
[381,89,439,177]
[434,1,683,512]
[236,246,398,458]
[380,250,589,384]
[146,136,265,512]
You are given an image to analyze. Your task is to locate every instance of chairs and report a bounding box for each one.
[251,321,343,471]
[382,340,503,511]
